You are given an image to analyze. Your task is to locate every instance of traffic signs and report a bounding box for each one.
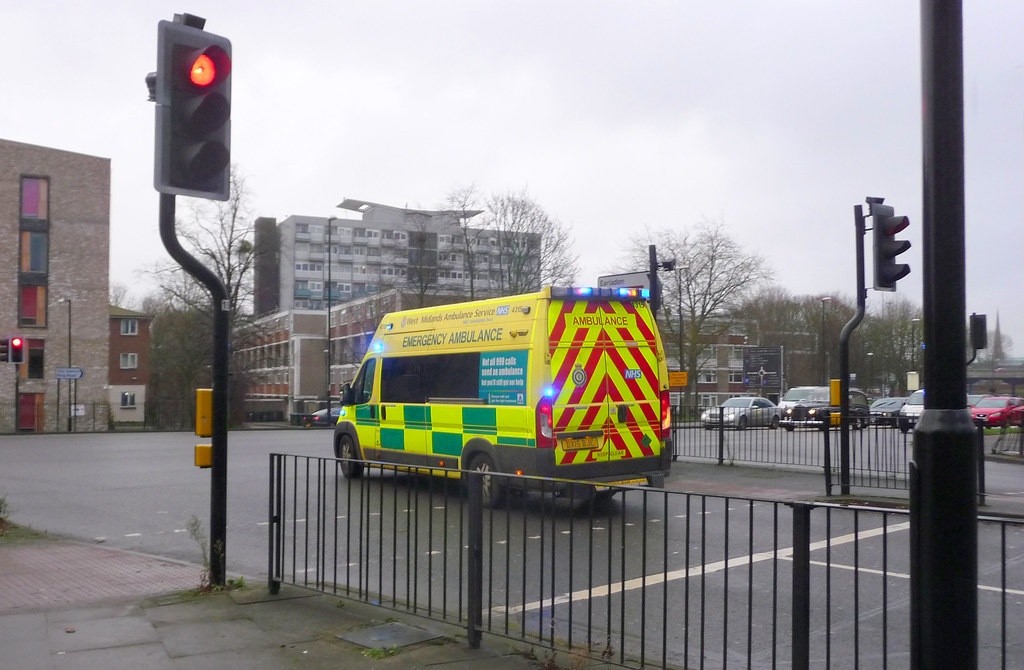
[741,346,784,389]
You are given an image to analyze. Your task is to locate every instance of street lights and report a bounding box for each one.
[910,318,921,372]
[57,298,73,431]
[328,214,338,426]
[647,258,689,319]
[866,352,874,389]
[821,296,834,386]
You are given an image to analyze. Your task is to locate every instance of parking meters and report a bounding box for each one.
[828,378,841,427]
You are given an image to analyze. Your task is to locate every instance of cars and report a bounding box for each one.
[700,396,782,431]
[967,393,995,409]
[898,389,927,432]
[970,396,1024,430]
[779,387,870,430]
[304,408,341,427]
[776,387,823,424]
[869,397,907,428]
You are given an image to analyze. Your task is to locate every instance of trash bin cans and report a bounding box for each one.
[290,413,306,425]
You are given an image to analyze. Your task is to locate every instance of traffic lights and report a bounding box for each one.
[0,338,8,364]
[871,201,911,293]
[7,336,25,365]
[155,18,234,202]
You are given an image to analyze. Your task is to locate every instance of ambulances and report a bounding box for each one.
[330,284,675,509]
